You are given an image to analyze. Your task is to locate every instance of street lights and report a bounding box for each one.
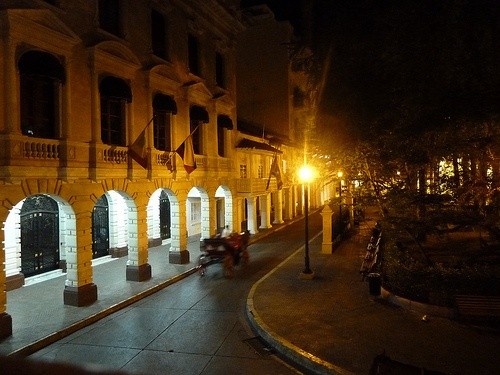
[299,167,315,280]
[336,169,344,240]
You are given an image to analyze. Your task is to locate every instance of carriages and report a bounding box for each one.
[197,229,251,278]
